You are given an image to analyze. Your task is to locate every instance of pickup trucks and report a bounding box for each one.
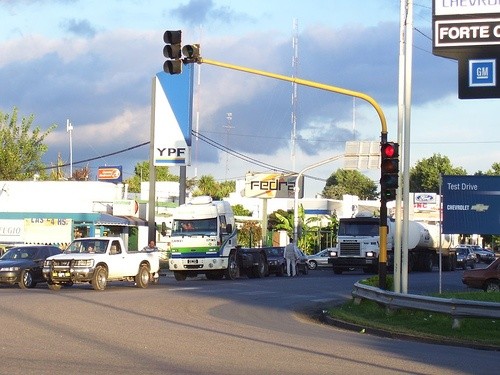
[41,236,160,291]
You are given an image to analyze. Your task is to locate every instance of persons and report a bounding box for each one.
[141,240,159,279]
[283,239,300,277]
[88,244,94,252]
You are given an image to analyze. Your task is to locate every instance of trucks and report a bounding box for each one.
[162,196,271,281]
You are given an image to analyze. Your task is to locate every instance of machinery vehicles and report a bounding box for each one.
[330,211,459,274]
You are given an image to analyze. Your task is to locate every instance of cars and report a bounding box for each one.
[306,247,337,271]
[0,244,63,288]
[462,257,500,294]
[454,244,497,270]
[262,246,309,277]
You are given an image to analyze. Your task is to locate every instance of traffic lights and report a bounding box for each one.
[182,42,200,59]
[163,30,182,75]
[380,141,399,191]
